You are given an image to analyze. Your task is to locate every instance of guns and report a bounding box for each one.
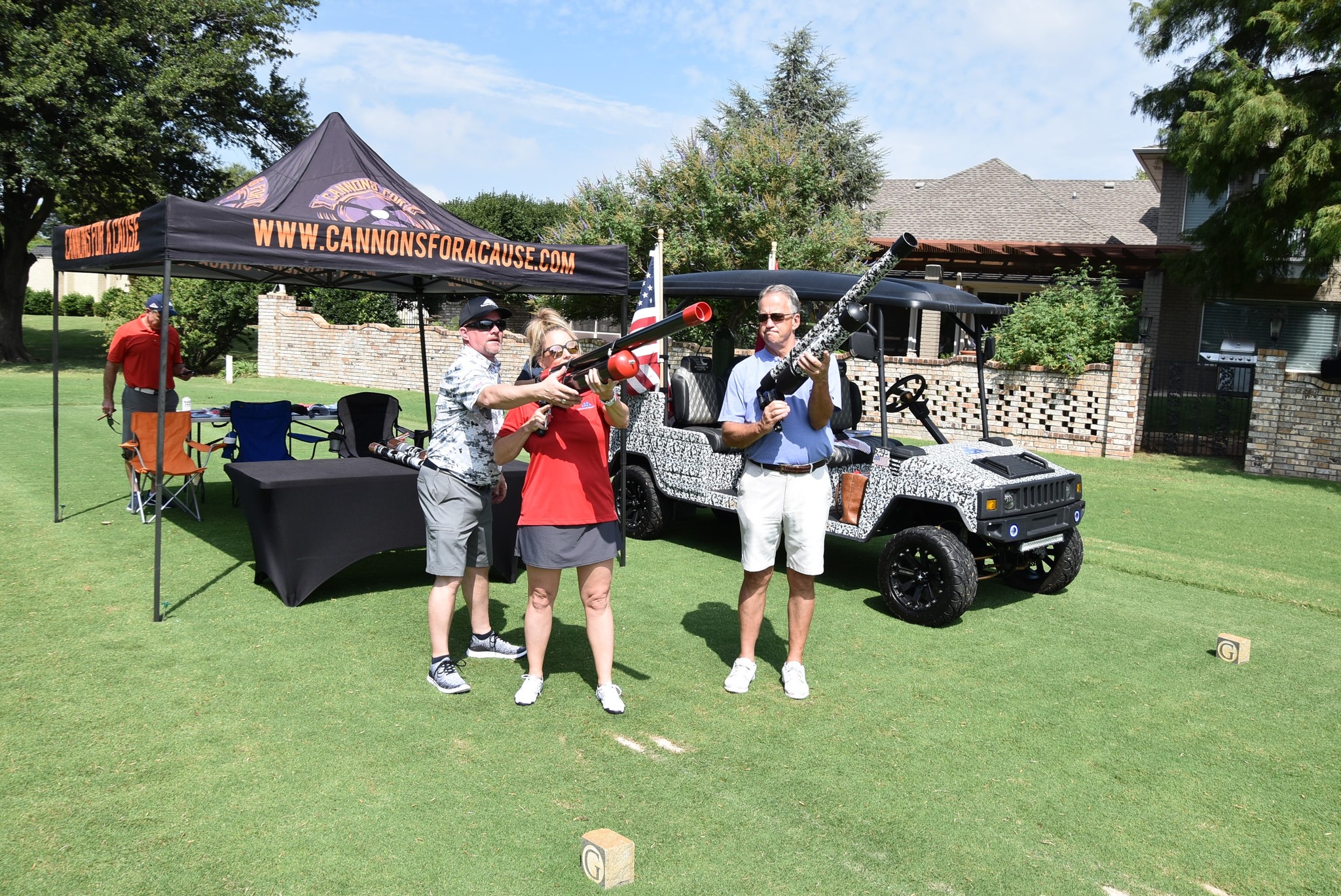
[756,229,920,433]
[535,300,713,438]
[368,431,429,469]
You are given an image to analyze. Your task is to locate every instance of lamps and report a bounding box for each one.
[1269,305,1285,350]
[1137,308,1153,343]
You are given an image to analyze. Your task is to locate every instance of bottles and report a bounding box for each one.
[182,397,191,411]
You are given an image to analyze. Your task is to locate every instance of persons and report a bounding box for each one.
[418,296,582,694]
[494,308,630,714]
[102,294,193,512]
[717,284,842,699]
[514,356,544,387]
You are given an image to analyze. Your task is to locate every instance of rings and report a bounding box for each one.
[562,399,566,404]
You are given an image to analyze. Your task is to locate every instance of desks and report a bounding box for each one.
[224,455,532,608]
[175,405,341,504]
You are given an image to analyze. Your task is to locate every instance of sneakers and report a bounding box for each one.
[126,492,144,511]
[426,656,471,694]
[723,656,757,693]
[146,490,174,508]
[782,660,810,700]
[595,681,625,714]
[513,673,544,705]
[466,630,528,659]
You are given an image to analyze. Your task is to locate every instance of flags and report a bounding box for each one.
[627,243,673,420]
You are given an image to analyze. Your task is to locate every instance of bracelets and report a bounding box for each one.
[599,384,621,407]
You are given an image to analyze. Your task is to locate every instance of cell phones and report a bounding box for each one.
[178,370,195,376]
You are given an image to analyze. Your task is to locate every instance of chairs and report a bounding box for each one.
[222,392,415,507]
[119,411,226,524]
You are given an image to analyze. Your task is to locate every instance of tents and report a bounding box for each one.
[50,112,630,623]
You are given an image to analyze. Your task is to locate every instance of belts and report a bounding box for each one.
[747,458,828,474]
[423,457,490,493]
[124,381,172,395]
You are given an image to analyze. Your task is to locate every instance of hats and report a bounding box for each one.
[146,293,181,318]
[459,296,512,328]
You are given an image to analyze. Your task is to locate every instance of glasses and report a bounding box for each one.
[540,339,580,359]
[463,319,506,331]
[757,313,796,322]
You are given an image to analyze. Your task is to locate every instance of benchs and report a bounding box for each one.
[672,355,750,453]
[827,360,904,466]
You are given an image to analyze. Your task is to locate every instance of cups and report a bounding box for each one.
[223,438,236,444]
